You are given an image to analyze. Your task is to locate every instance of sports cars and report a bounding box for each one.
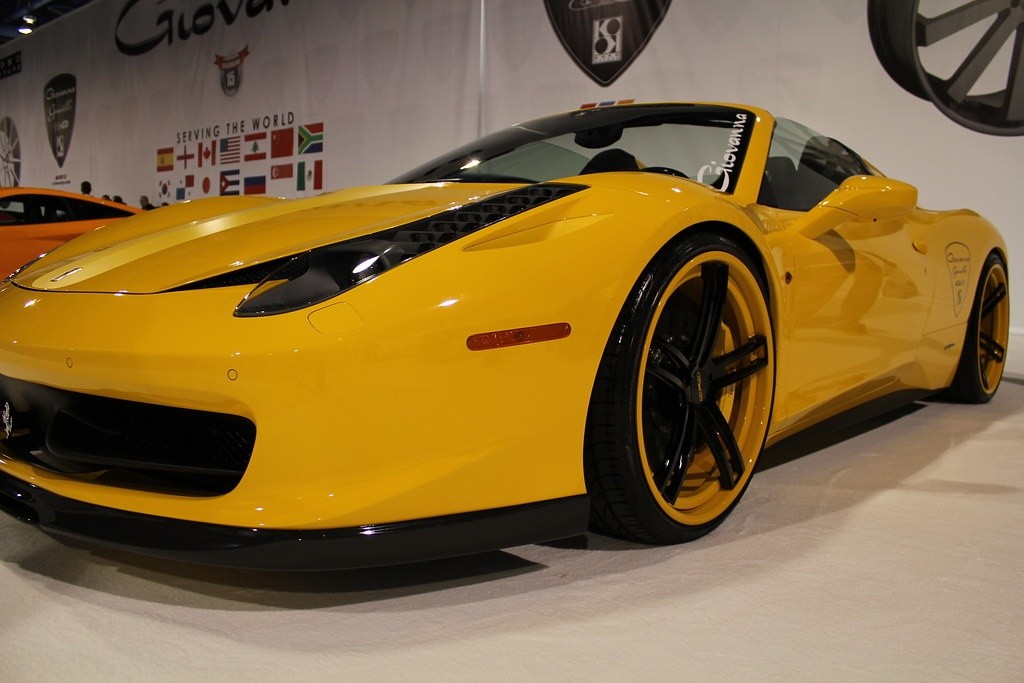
[0,99,1012,576]
[1,186,148,283]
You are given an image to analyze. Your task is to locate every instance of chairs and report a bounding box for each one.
[579,148,641,176]
[757,157,797,210]
